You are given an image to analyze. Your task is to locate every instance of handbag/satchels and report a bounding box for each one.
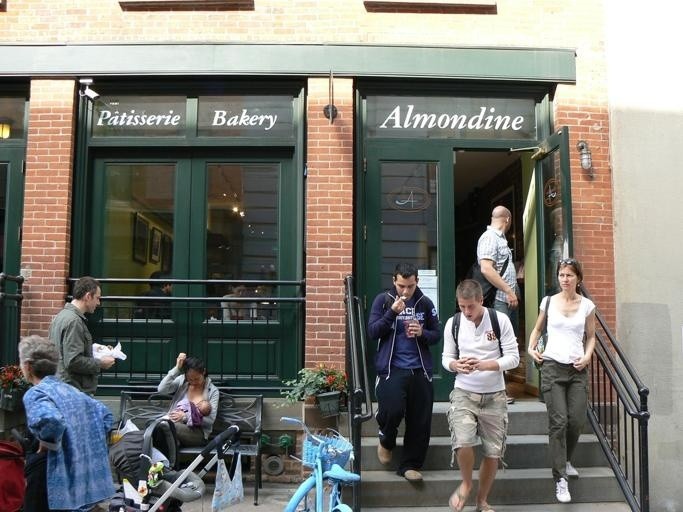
[466,261,496,307]
[211,451,245,512]
[532,328,548,369]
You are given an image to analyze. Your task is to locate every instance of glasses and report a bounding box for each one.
[558,258,576,266]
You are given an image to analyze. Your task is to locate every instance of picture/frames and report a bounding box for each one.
[161,233,173,276]
[130,212,150,266]
[150,226,164,264]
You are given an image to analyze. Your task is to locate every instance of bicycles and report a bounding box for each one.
[279,416,361,512]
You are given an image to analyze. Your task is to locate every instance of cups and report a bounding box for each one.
[401,320,416,338]
[110,430,122,445]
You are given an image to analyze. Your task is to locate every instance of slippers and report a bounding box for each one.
[476,505,495,512]
[448,481,473,512]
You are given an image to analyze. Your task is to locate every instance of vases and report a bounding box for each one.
[312,389,343,420]
[1,392,27,414]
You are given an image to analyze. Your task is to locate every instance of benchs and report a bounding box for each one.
[105,390,265,506]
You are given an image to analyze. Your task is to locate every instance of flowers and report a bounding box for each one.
[271,360,351,408]
[1,363,32,396]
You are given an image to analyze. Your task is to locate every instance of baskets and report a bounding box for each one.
[301,428,354,472]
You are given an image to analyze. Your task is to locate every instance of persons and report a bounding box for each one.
[18,335,116,511]
[527,258,596,503]
[132,271,266,321]
[48,277,114,399]
[151,351,223,471]
[366,261,442,483]
[477,204,520,405]
[442,280,520,512]
[172,400,212,429]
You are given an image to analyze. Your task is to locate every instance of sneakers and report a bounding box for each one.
[377,443,393,465]
[506,395,515,404]
[555,477,571,503]
[565,462,579,476]
[404,469,423,481]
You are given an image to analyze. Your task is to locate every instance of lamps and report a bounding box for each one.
[575,140,591,169]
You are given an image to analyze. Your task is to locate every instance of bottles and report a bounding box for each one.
[532,337,544,369]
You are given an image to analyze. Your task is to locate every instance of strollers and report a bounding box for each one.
[108,418,241,512]
